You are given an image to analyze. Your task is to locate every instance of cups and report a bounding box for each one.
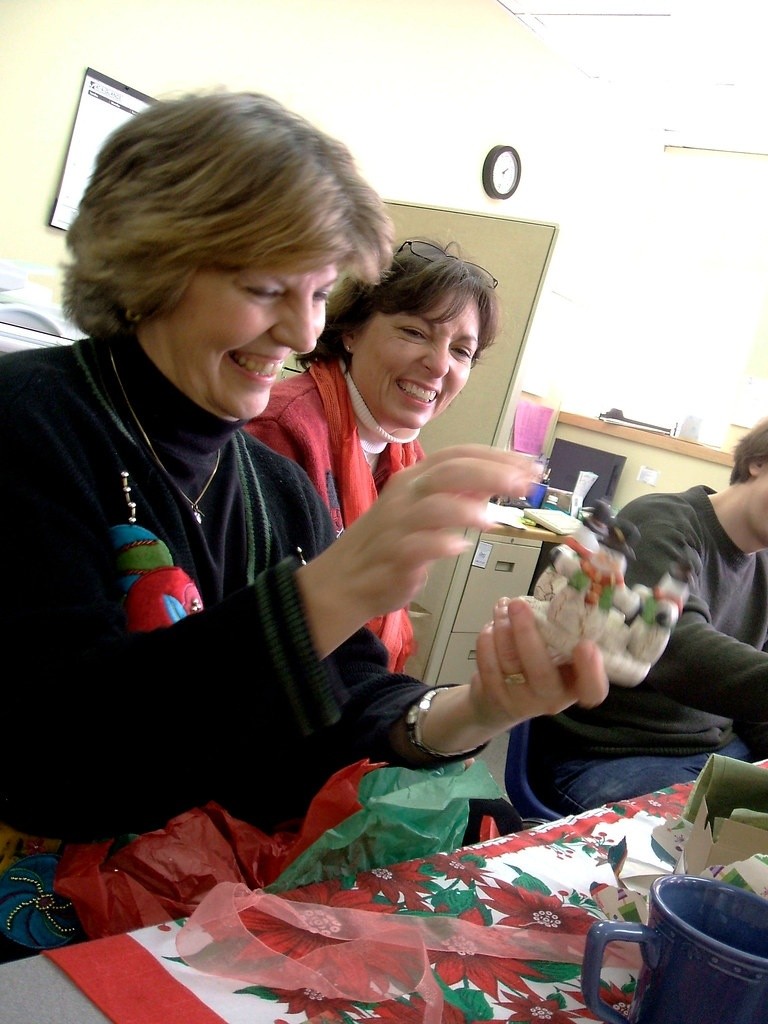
[526,482,548,508]
[582,874,768,1024]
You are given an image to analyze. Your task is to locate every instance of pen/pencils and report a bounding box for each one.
[540,468,552,485]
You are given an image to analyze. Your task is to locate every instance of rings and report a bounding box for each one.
[504,670,527,683]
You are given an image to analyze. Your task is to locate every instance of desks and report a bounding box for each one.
[0,759,768,1024]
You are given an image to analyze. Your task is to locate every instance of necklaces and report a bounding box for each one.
[109,347,221,524]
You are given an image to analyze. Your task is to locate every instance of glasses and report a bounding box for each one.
[395,240,498,293]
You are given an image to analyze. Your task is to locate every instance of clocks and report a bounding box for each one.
[482,144,522,200]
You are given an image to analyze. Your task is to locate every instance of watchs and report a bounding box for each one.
[407,687,480,759]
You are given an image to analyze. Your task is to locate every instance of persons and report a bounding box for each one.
[527,416,768,814]
[0,90,610,967]
[241,238,500,675]
